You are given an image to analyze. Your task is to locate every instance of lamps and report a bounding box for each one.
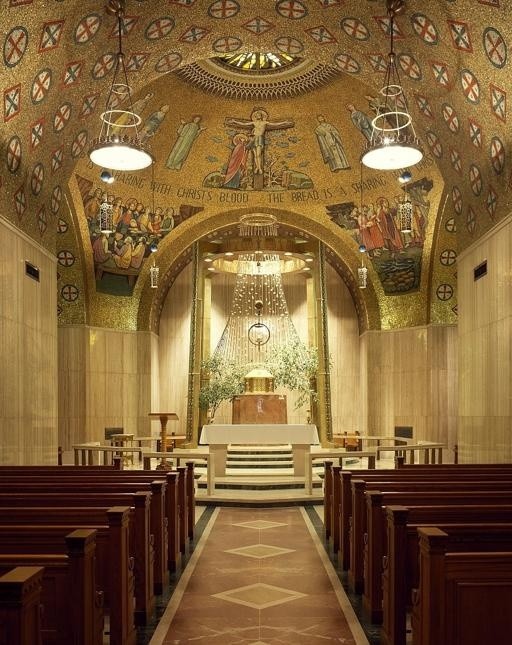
[358,0,424,289]
[87,0,159,289]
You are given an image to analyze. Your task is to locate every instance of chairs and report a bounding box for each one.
[324,456,512,645]
[0,456,196,645]
